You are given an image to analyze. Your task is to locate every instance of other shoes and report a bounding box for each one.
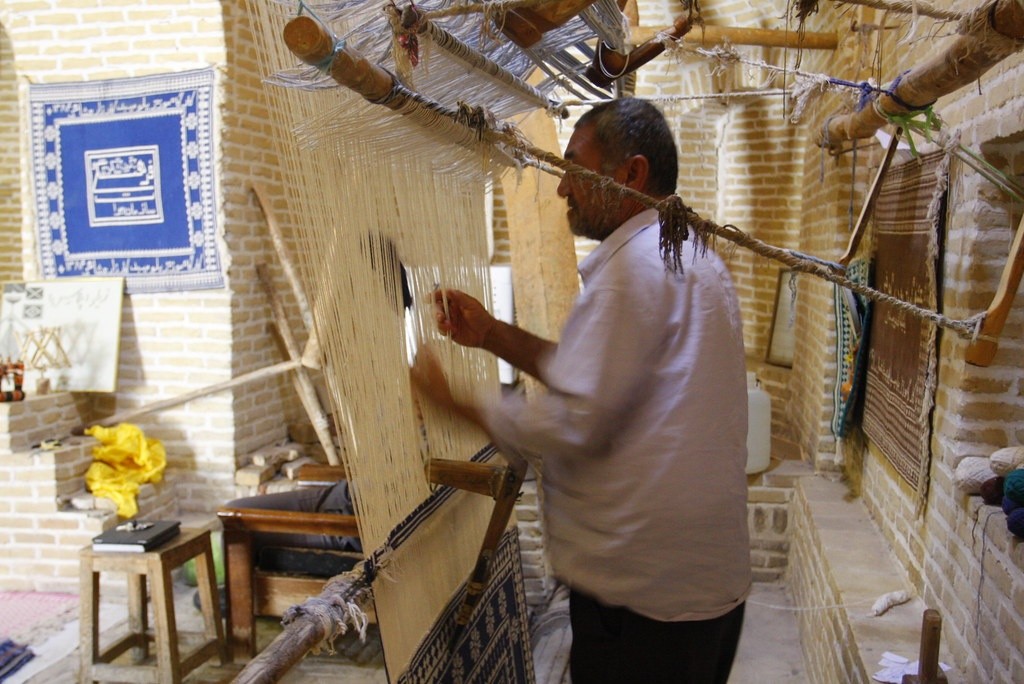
[193,588,228,618]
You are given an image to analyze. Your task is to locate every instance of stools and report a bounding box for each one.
[76,526,229,684]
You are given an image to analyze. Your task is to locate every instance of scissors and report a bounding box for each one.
[26,438,63,457]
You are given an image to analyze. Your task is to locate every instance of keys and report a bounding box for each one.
[116,519,153,531]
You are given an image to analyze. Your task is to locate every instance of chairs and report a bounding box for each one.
[214,462,377,668]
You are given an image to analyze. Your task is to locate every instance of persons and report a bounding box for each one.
[409,97,752,684]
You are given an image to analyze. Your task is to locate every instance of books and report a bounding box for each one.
[92,518,181,553]
